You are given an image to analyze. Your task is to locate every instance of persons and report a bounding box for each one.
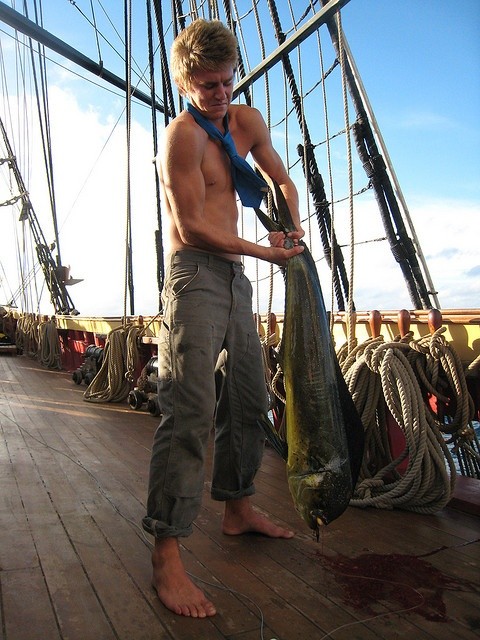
[142,20,305,618]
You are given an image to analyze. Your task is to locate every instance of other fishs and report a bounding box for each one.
[255,178,367,532]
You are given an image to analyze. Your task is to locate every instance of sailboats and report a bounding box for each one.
[0,0,480,640]
